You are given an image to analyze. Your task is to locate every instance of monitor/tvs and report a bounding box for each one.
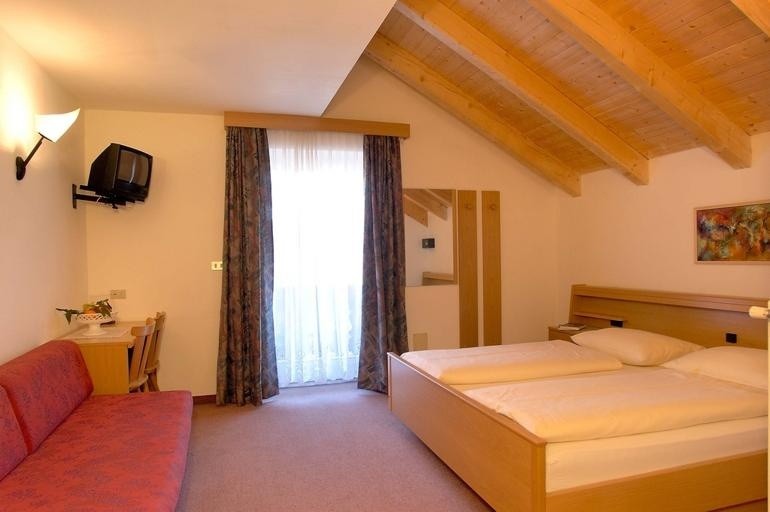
[87,141,152,202]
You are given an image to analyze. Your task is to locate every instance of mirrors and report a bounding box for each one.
[403,187,458,289]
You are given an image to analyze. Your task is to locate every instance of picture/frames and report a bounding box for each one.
[693,200,770,265]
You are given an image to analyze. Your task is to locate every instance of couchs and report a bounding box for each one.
[0,339,194,511]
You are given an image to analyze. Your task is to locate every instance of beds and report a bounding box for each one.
[387,285,770,512]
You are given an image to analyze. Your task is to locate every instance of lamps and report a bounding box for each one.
[16,108,81,181]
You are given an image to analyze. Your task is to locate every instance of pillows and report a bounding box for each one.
[570,327,708,366]
[658,345,769,389]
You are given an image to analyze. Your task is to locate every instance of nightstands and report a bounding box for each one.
[548,322,603,343]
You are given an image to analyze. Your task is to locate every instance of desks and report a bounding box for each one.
[54,321,147,393]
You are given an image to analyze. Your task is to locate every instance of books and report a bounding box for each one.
[560,323,586,330]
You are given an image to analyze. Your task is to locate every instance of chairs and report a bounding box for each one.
[129,316,157,393]
[146,312,167,393]
